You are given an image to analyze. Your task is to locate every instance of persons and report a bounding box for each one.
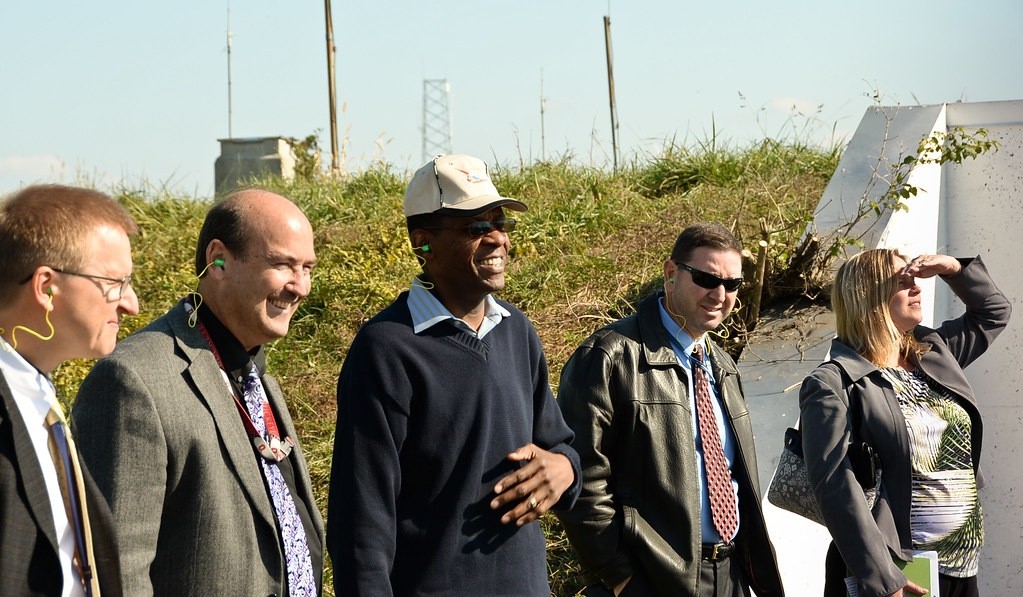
[69,185,327,597]
[549,223,787,597]
[326,152,584,597]
[799,248,1013,597]
[0,184,141,597]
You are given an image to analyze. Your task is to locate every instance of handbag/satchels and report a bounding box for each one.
[767,361,882,529]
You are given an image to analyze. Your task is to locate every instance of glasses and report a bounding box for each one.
[672,258,745,292]
[17,267,137,299]
[426,217,517,236]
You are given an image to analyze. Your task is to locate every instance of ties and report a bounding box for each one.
[45,407,100,597]
[691,344,738,545]
[241,360,317,597]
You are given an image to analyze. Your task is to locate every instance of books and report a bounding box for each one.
[847,549,940,597]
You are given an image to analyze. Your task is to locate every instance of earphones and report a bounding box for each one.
[420,242,432,253]
[669,278,674,284]
[46,288,53,297]
[213,258,225,271]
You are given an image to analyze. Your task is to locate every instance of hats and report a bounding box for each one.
[403,156,528,218]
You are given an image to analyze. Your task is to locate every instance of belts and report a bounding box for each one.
[702,532,741,561]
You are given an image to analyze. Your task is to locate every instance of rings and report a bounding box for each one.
[921,259,929,262]
[528,496,538,508]
